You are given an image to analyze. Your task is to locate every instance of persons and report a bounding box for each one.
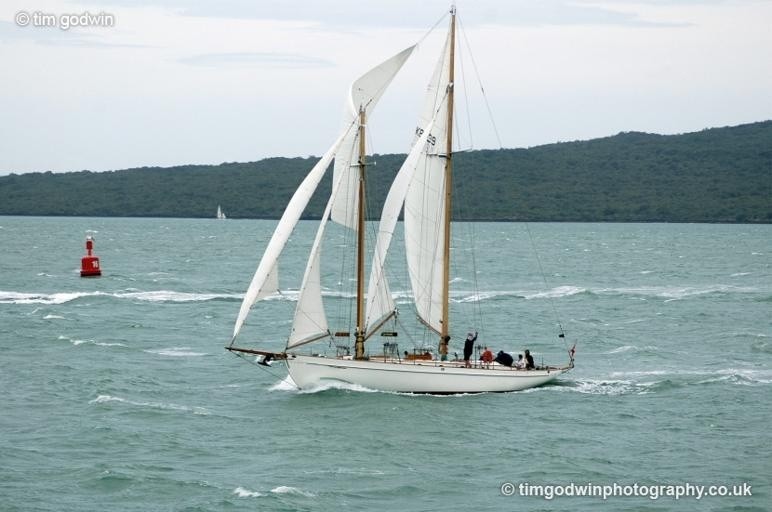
[480,347,535,370]
[463,331,478,368]
[439,336,451,361]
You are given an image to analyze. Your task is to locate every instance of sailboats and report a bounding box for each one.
[214,0,579,396]
[215,203,227,220]
